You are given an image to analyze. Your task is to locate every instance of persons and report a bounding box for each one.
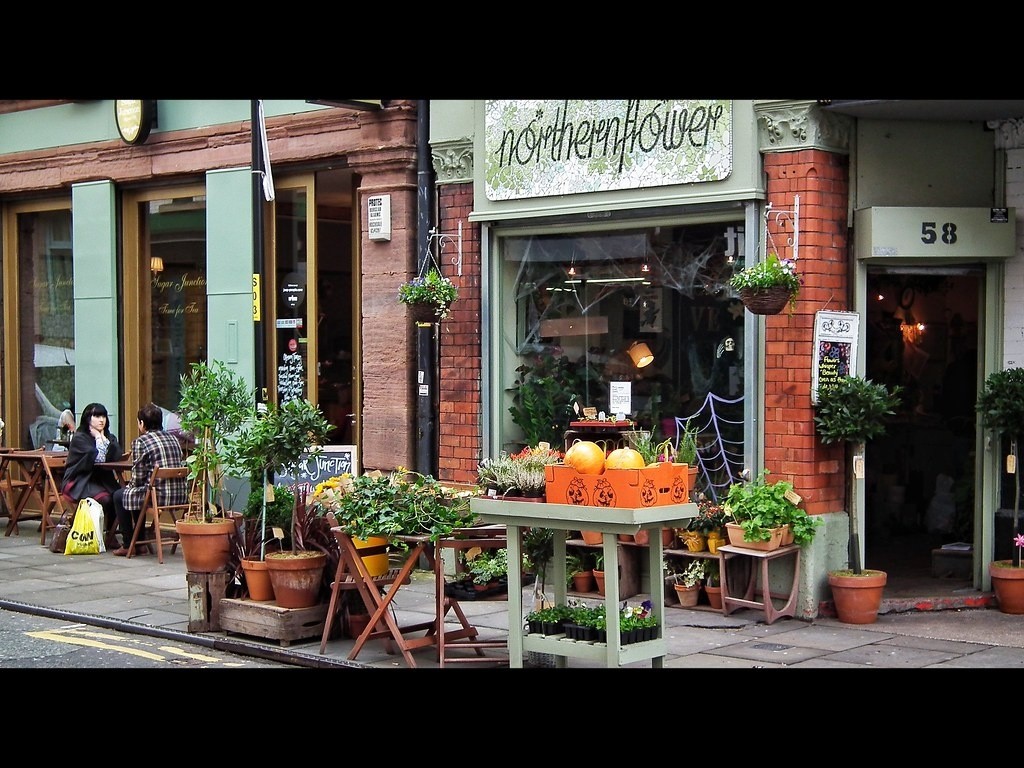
[51,391,75,451]
[113,404,189,557]
[61,402,132,548]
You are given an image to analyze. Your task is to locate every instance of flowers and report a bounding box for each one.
[525,599,657,633]
[475,448,564,492]
[313,474,350,500]
[664,502,733,609]
[397,271,461,337]
[730,259,804,319]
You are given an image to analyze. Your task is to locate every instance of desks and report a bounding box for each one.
[0,453,69,545]
[95,459,152,556]
[319,525,484,667]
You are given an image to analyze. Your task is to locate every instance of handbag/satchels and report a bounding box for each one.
[64,497,107,557]
[48,508,75,554]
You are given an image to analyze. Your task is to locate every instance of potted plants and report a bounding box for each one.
[444,546,535,591]
[565,550,607,597]
[674,366,1024,624]
[173,360,471,639]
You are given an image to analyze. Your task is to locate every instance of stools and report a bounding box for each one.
[718,545,801,622]
[932,549,974,579]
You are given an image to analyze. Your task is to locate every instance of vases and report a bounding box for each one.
[527,621,657,644]
[486,485,542,499]
[409,300,451,323]
[739,286,790,316]
[578,528,728,608]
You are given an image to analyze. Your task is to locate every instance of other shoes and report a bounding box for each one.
[112,544,137,558]
[137,544,151,557]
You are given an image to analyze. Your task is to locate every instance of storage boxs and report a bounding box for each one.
[545,463,690,509]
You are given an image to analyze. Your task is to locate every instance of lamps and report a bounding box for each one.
[150,257,165,277]
[629,343,654,368]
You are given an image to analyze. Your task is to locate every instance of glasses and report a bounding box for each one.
[608,371,634,381]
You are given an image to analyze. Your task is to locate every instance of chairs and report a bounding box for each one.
[0,446,197,563]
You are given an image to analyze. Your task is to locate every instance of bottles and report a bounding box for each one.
[55,426,61,440]
[67,430,71,442]
[70,431,74,442]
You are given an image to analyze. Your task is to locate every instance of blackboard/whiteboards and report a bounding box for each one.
[273,445,359,495]
[811,310,860,405]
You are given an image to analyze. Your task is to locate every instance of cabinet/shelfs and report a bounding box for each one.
[470,497,699,668]
